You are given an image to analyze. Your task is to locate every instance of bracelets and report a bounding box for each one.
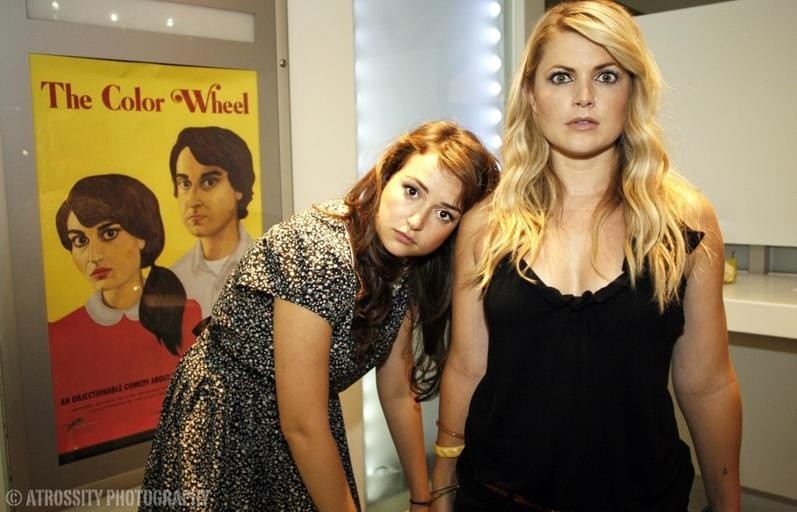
[431,442,465,458]
[436,420,464,439]
[409,498,428,505]
[430,481,459,495]
[426,485,459,505]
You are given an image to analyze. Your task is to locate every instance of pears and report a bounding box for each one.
[723,250,738,283]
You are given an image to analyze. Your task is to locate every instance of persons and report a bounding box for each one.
[132,119,501,512]
[163,125,256,321]
[44,174,202,454]
[426,0,743,511]
